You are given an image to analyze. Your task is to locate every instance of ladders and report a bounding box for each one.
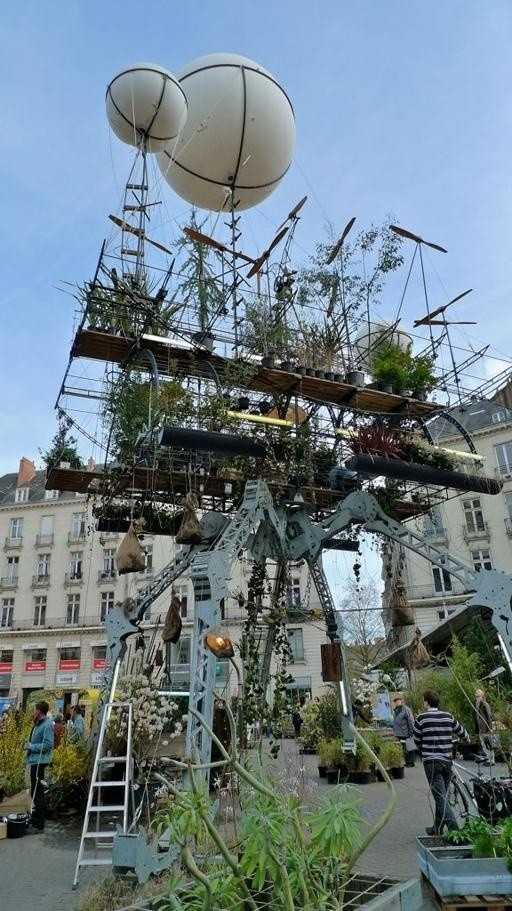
[71,703,136,890]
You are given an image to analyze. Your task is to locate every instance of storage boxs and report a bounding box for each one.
[138,731,186,758]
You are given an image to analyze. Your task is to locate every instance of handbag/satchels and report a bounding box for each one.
[405,735,418,751]
[484,733,501,749]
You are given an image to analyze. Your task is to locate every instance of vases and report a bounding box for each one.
[457,741,478,761]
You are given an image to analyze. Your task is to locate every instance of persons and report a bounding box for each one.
[228,693,321,737]
[390,697,416,768]
[27,698,87,831]
[2,706,14,721]
[414,691,471,835]
[470,690,494,767]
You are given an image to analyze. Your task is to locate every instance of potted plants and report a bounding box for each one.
[41,378,459,536]
[56,207,436,403]
[297,693,406,785]
[414,812,512,897]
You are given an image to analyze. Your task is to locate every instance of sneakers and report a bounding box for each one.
[426,826,444,835]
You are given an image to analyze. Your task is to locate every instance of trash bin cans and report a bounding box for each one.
[472,777,512,827]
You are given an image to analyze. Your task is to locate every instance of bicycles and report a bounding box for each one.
[450,739,512,831]
